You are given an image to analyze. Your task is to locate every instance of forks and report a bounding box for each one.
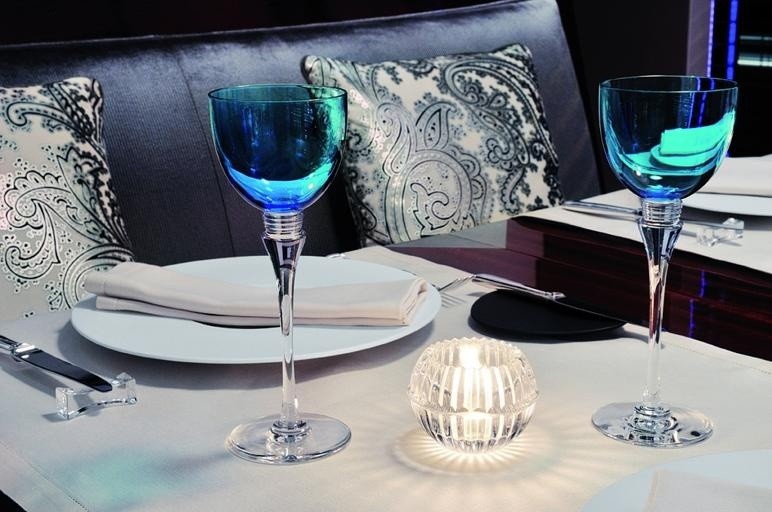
[430,274,478,293]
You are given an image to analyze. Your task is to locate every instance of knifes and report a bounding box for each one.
[473,272,646,328]
[563,199,745,233]
[0,336,113,396]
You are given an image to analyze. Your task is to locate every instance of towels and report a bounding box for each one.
[700,155,772,196]
[83,261,428,327]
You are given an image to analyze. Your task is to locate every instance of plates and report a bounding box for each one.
[70,253,443,367]
[682,191,771,216]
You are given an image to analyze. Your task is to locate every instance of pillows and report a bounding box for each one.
[0,78,135,314]
[301,42,563,248]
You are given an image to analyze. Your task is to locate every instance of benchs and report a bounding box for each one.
[0,0,603,264]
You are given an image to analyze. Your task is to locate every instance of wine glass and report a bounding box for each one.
[207,83,349,465]
[589,75,738,448]
